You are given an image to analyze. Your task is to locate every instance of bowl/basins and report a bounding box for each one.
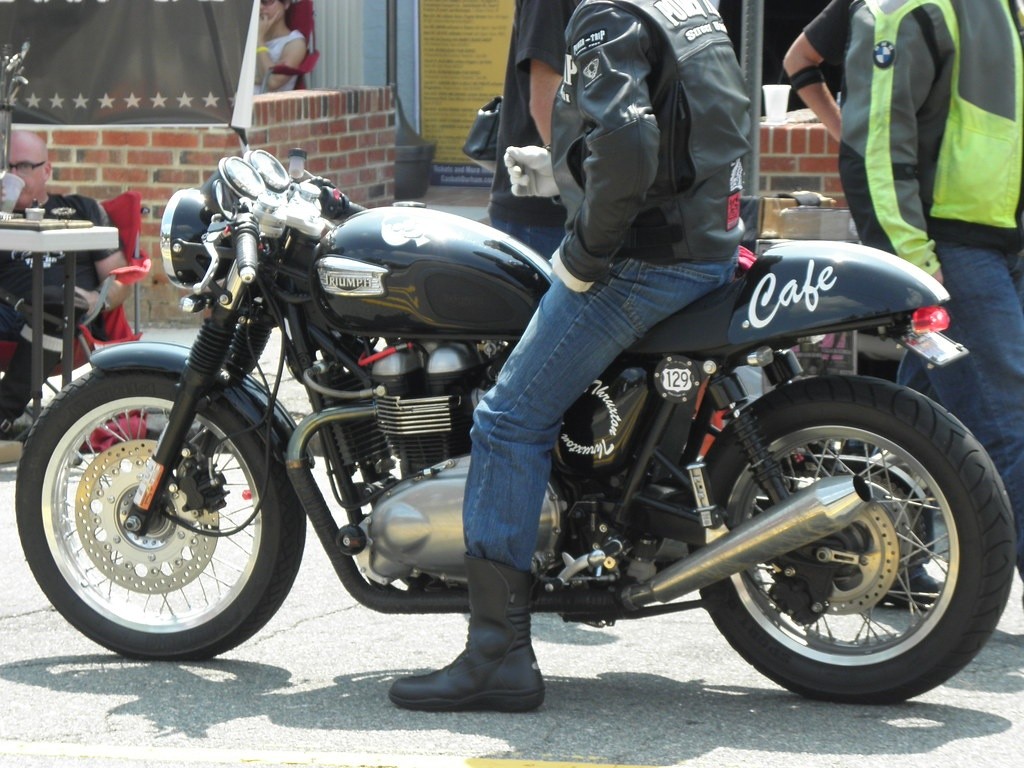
[24,207,45,222]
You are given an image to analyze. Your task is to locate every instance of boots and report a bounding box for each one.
[389,553,545,714]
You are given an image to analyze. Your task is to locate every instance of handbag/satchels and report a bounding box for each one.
[463,96,503,173]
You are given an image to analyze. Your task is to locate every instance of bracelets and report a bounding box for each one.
[257,46,269,52]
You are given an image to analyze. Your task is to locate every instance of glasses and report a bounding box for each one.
[10,161,44,173]
[261,0,275,6]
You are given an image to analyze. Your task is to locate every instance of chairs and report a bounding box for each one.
[0,190,154,403]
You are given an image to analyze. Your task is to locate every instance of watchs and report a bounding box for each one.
[91,289,112,312]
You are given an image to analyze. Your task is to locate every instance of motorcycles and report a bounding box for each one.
[13,122,1018,706]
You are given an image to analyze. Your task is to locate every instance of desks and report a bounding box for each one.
[0,223,121,422]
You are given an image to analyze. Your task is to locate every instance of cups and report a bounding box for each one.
[0,173,26,217]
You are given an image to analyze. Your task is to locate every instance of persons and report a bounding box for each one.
[253,0,306,94]
[838,0,1024,612]
[391,1,752,712]
[783,0,853,145]
[0,132,133,466]
[488,1,584,259]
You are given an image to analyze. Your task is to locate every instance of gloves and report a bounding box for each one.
[504,145,561,197]
[550,248,594,292]
